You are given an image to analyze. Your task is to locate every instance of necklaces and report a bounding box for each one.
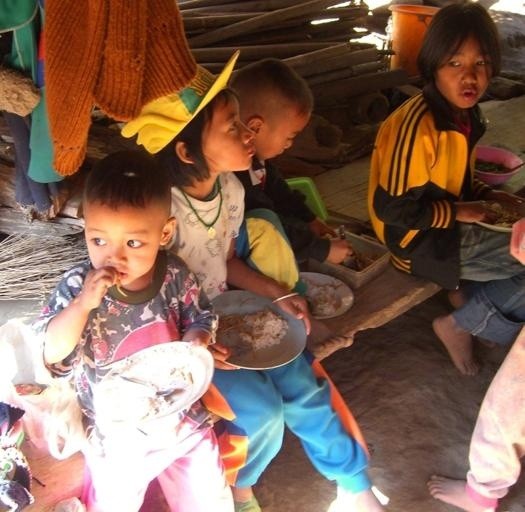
[177,178,224,239]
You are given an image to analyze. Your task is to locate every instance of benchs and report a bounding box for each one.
[20,95,524,512]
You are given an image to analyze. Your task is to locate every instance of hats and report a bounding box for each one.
[121,50,240,154]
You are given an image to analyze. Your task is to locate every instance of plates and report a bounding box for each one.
[94,341,214,422]
[471,200,523,232]
[296,270,355,319]
[207,288,308,372]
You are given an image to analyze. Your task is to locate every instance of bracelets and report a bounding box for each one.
[271,292,299,304]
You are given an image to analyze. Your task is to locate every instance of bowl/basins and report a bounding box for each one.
[471,143,523,187]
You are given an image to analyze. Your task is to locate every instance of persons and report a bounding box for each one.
[368,2,524,374]
[229,59,386,511]
[124,49,248,511]
[34,150,234,511]
[428,218,525,512]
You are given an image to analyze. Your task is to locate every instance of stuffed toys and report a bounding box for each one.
[0,67,41,121]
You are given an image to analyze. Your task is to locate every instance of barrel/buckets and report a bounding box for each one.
[389,4,449,82]
